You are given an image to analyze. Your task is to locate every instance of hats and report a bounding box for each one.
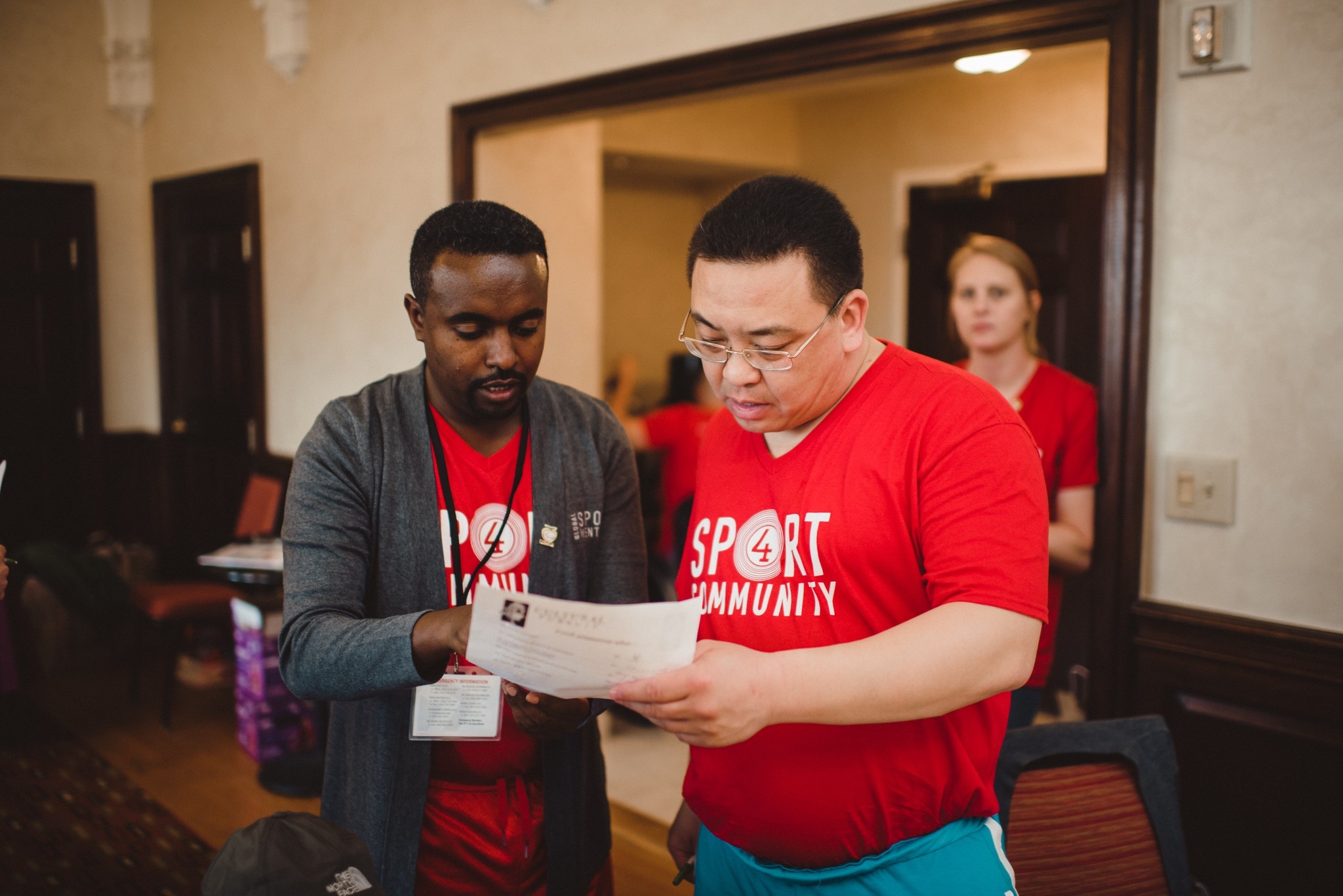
[204,808,380,896]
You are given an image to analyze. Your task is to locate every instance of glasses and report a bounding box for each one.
[679,294,845,372]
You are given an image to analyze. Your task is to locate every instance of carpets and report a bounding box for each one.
[0,693,217,896]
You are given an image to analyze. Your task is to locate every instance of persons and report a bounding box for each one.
[278,200,650,896]
[602,172,1098,896]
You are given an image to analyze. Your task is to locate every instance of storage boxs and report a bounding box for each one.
[229,597,318,757]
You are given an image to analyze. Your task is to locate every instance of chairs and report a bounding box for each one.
[128,454,292,728]
[995,716,1188,896]
